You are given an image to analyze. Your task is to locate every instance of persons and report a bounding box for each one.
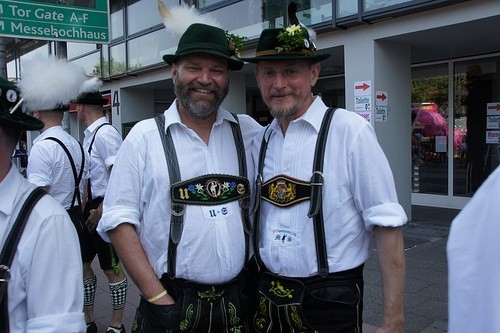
[445,165,500,333]
[0,76,87,333]
[95,23,266,333]
[236,23,409,333]
[17,55,127,333]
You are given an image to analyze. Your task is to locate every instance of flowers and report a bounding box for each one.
[274,23,307,53]
[226,30,247,58]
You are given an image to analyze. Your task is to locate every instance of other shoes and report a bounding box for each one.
[86,321,98,333]
[106,323,126,333]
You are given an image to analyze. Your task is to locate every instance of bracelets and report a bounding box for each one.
[147,289,167,303]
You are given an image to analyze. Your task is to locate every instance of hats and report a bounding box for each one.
[0,75,45,130]
[53,91,108,111]
[239,28,331,62]
[162,23,245,71]
